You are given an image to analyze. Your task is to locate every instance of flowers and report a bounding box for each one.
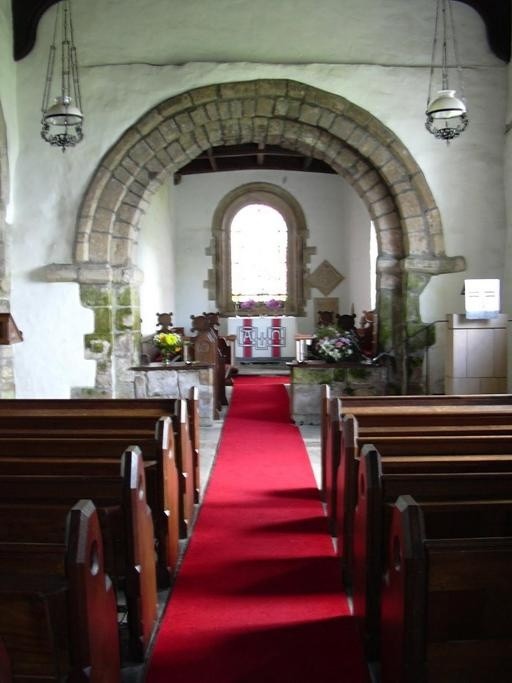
[153,332,182,355]
[314,323,353,361]
[240,299,280,312]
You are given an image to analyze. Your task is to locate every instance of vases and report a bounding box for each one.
[163,355,170,366]
[325,359,337,362]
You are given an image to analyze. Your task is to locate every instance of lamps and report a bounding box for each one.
[425,0,469,141]
[41,0,83,152]
